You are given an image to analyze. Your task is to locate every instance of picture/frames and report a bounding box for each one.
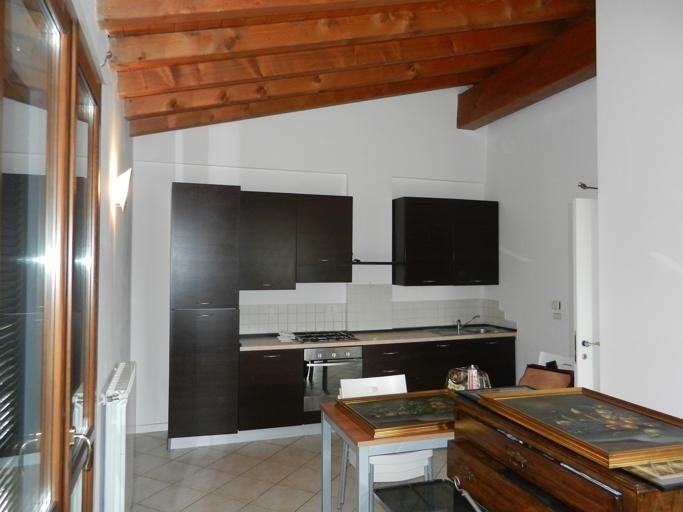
[620,460,682,492]
[337,388,460,440]
[466,386,682,468]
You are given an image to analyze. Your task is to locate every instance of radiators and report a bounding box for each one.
[100,362,138,512]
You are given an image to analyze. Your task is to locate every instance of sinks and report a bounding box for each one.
[462,325,498,334]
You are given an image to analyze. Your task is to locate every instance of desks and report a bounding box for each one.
[319,400,455,512]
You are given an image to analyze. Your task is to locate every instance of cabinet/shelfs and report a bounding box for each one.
[166,182,238,447]
[238,188,352,290]
[445,396,683,511]
[390,196,499,286]
[238,349,304,444]
[362,336,515,393]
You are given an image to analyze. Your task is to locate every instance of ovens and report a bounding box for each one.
[304,347,364,412]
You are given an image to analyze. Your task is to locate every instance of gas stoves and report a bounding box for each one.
[292,331,362,342]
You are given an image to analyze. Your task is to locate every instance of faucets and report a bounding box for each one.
[457,314,481,336]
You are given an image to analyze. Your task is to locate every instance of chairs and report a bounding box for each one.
[334,372,434,512]
